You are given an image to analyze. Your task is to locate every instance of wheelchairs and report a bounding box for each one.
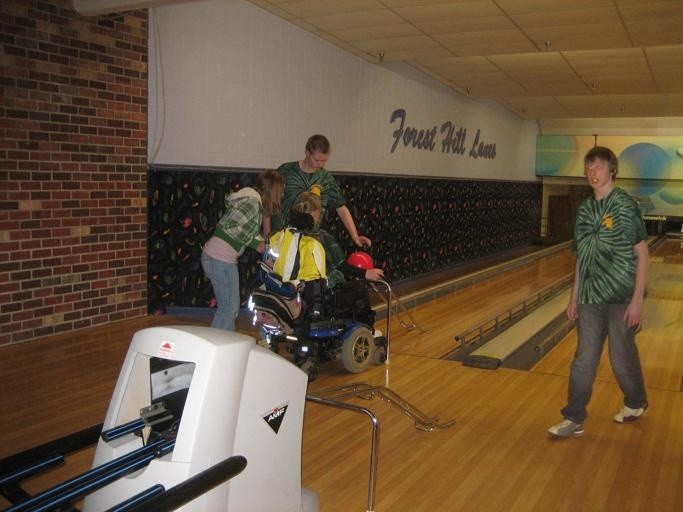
[252,224,390,382]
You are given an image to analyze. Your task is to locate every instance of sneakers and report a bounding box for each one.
[611,400,650,423]
[547,418,585,438]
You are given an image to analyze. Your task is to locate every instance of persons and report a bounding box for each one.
[261,134,373,249]
[546,146,650,437]
[288,191,384,339]
[198,167,287,333]
